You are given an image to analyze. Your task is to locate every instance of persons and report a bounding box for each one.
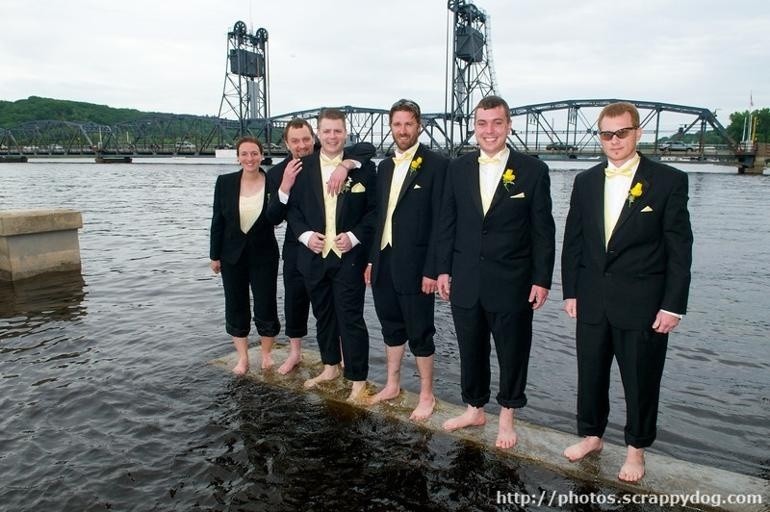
[435,95,554,448]
[267,110,375,404]
[365,100,448,424]
[563,101,692,481]
[211,137,280,374]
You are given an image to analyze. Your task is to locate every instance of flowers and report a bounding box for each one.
[627,182,644,207]
[339,175,354,194]
[408,156,422,177]
[502,167,516,190]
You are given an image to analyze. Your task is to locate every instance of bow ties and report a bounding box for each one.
[392,152,412,166]
[604,168,632,177]
[478,157,502,165]
[325,158,340,166]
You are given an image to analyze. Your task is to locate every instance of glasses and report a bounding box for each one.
[392,101,419,115]
[599,128,634,140]
